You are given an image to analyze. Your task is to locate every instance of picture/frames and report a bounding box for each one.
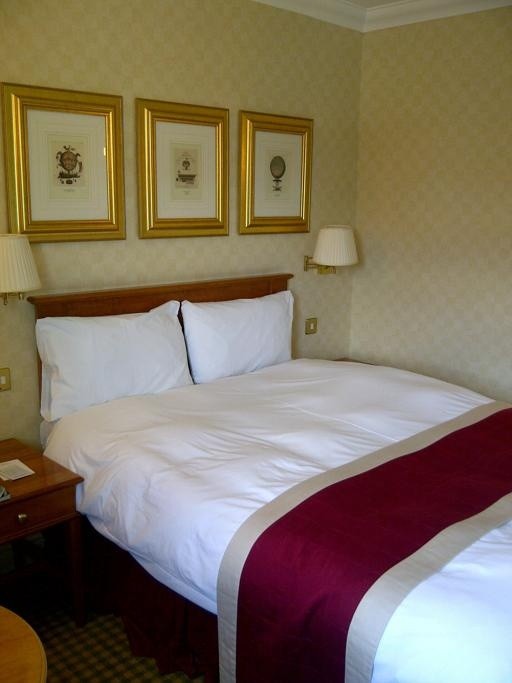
[0,607,47,683]
[0,438,85,630]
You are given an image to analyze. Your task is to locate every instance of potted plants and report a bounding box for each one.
[238,110,314,236]
[135,97,231,239]
[0,82,126,242]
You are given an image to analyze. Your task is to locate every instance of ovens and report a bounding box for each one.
[25,273,512,682]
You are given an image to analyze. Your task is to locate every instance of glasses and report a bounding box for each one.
[304,225,360,275]
[0,232,45,307]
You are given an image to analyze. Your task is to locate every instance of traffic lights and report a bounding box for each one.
[181,290,295,384]
[35,301,195,422]
